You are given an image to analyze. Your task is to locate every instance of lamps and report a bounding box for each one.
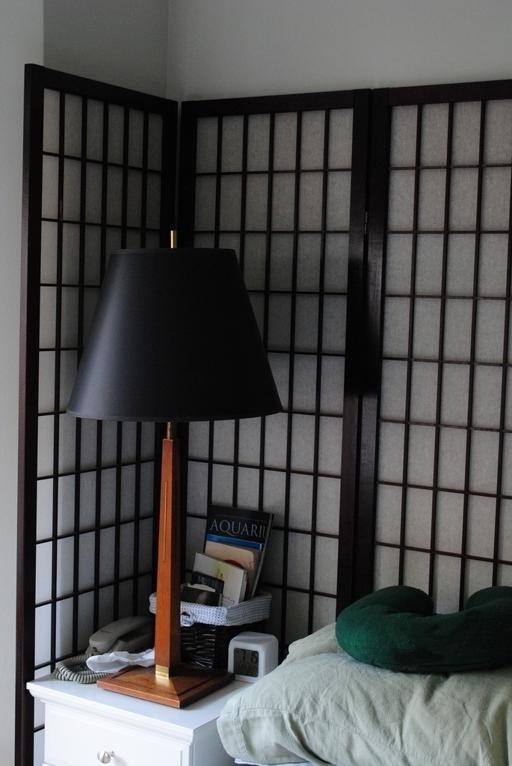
[66,243,292,713]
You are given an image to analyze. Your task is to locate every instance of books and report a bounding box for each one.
[181,503,275,607]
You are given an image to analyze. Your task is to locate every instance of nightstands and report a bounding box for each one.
[26,666,261,764]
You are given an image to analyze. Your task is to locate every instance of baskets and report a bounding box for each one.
[148,582,273,671]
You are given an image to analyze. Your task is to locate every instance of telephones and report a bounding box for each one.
[85,616,155,659]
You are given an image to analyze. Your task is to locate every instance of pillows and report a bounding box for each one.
[335,582,509,678]
